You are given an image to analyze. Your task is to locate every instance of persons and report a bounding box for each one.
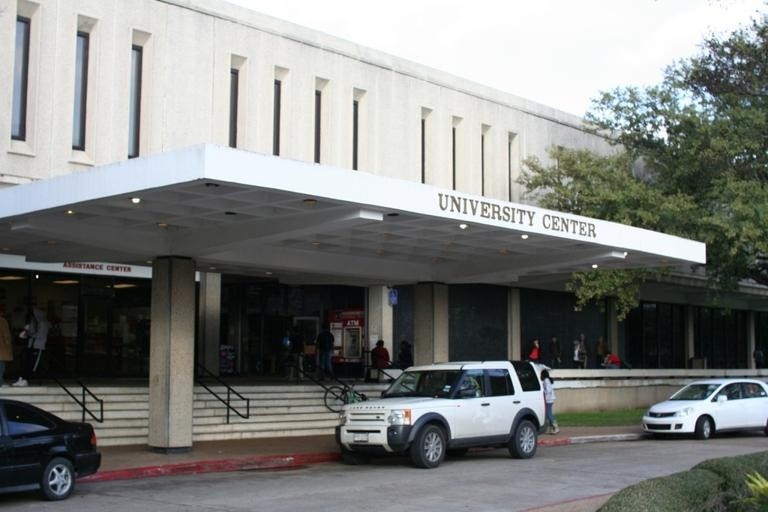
[529,333,621,434]
[0,303,50,388]
[278,321,413,386]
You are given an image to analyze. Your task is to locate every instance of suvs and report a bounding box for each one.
[336,360,550,468]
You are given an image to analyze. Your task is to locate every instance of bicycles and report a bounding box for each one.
[324,381,369,414]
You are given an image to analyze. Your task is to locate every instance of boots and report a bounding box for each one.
[545,426,553,434]
[552,422,559,434]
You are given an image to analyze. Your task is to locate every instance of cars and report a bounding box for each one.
[0,398,104,502]
[643,378,768,440]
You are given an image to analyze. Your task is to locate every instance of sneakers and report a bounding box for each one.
[12,376,28,387]
